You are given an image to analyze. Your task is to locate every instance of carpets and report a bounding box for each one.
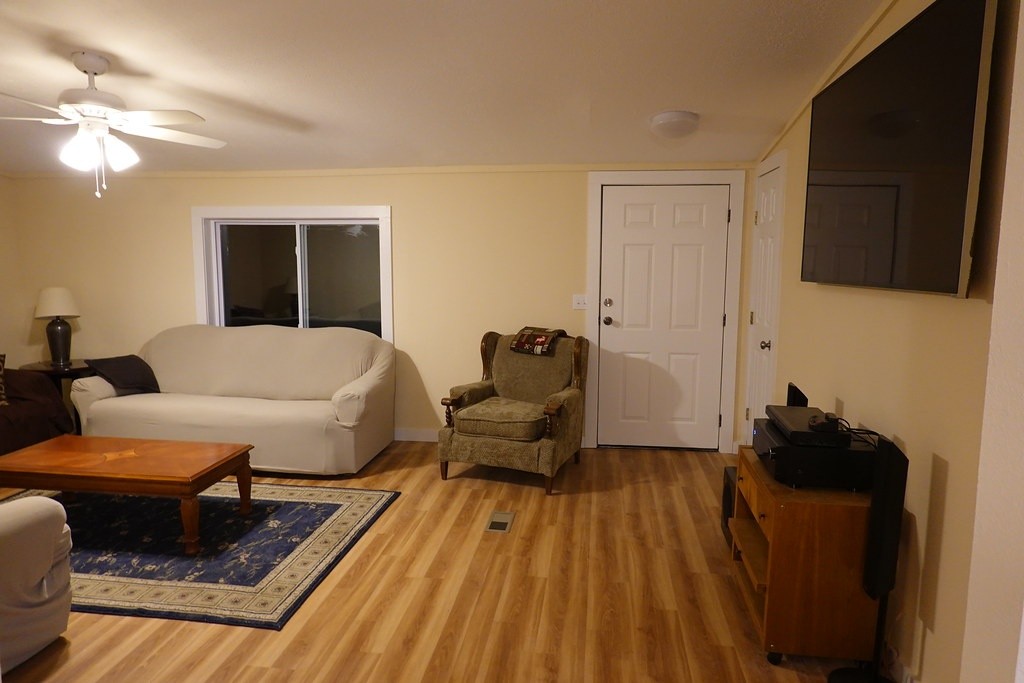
[1,478,402,630]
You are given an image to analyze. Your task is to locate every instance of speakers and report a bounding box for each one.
[721,465,737,550]
[827,435,909,683]
[786,382,809,407]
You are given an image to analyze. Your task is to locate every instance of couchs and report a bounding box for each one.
[0,496,73,675]
[440,329,590,493]
[77,324,395,479]
[0,369,69,458]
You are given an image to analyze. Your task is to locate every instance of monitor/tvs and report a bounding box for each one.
[800,0,998,298]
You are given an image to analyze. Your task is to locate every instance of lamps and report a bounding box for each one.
[650,110,700,139]
[58,117,141,182]
[32,288,80,369]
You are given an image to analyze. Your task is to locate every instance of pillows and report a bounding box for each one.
[0,354,9,406]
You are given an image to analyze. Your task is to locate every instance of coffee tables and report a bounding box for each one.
[0,434,255,556]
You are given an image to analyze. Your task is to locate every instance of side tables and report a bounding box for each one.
[20,358,92,434]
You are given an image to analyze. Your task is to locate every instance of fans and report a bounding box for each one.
[0,51,225,150]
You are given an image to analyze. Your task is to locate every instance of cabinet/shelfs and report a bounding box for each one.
[724,440,887,665]
